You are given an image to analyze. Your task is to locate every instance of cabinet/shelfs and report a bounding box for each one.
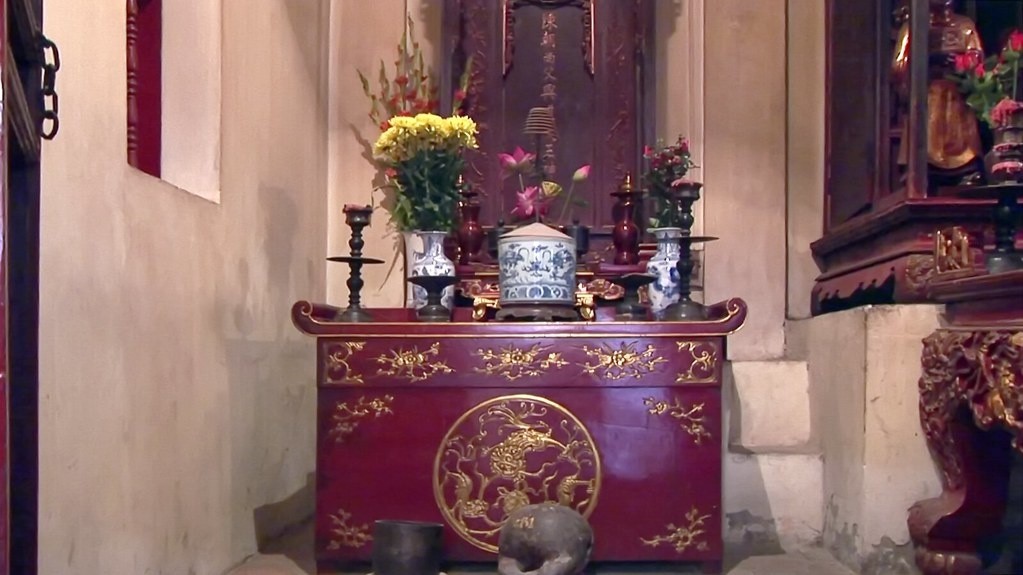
[291,298,747,574]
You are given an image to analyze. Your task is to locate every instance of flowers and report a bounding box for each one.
[356,12,481,233]
[497,145,590,221]
[639,133,701,227]
[945,29,1023,129]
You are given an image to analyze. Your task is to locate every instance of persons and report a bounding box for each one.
[891,0,987,188]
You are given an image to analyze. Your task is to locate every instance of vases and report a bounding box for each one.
[400,228,457,317]
[985,113,1023,182]
[645,228,681,320]
